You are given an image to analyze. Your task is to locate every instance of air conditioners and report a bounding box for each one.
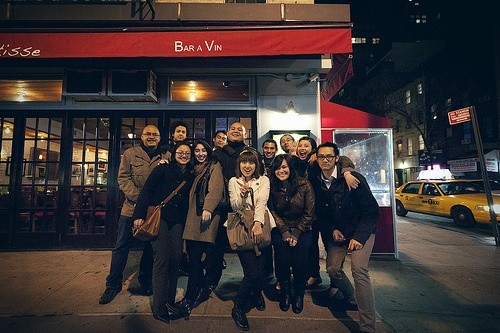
[62,67,114,102]
[107,68,159,103]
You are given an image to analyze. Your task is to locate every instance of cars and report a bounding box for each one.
[394,165,500,228]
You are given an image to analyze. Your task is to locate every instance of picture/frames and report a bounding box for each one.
[98,158,107,171]
[5,156,27,177]
[72,165,81,177]
[88,161,95,177]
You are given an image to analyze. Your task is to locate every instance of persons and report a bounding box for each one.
[133,120,380,333]
[98,125,171,305]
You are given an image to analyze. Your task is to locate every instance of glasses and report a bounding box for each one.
[142,133,160,138]
[318,155,336,162]
[175,152,191,156]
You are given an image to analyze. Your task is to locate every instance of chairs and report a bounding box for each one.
[19,191,106,234]
[410,188,417,193]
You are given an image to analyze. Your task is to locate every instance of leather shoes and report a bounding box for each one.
[179,262,191,278]
[192,285,209,308]
[141,280,153,296]
[221,259,226,268]
[165,297,191,319]
[279,289,291,311]
[231,303,249,331]
[292,291,304,313]
[154,313,170,324]
[98,286,122,304]
[256,293,266,311]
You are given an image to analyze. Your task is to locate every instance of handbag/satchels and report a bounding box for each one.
[228,187,271,256]
[132,205,161,241]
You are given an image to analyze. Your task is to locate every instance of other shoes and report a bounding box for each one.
[274,280,280,294]
[306,276,322,287]
[322,285,339,299]
[329,300,358,311]
